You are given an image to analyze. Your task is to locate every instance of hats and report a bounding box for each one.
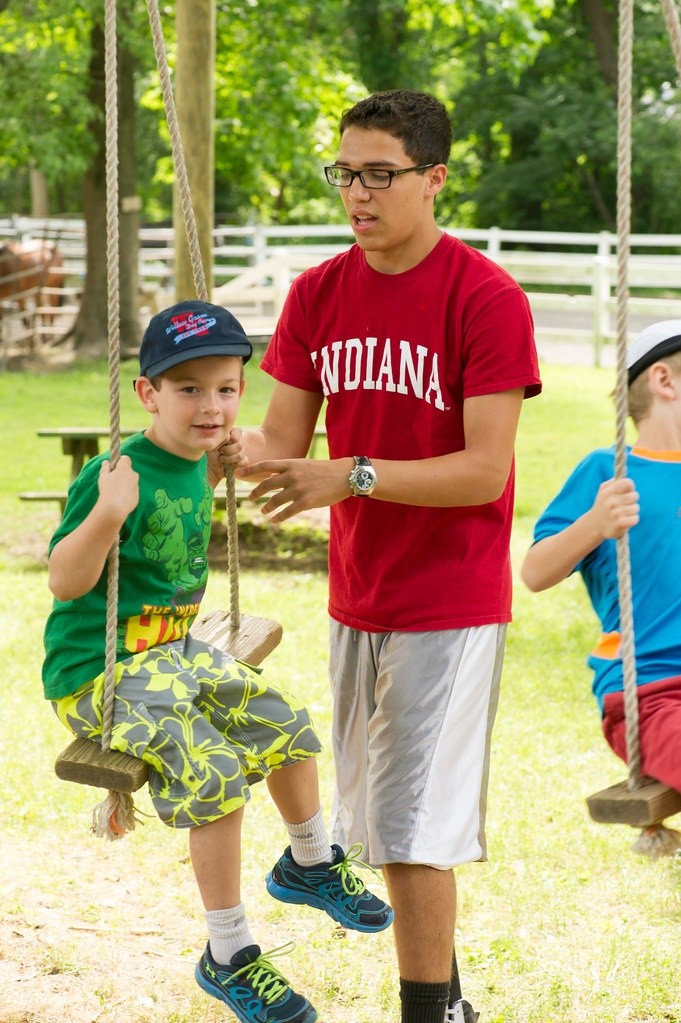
[627,318,680,384]
[132,301,254,391]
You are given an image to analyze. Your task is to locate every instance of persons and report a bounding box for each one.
[44,297,398,1021]
[521,319,680,799]
[226,92,546,1020]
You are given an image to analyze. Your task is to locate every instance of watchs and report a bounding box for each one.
[346,455,377,497]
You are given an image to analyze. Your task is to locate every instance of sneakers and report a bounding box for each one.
[265,844,394,935]
[194,940,317,1023]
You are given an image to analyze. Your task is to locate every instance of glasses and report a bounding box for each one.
[324,163,433,189]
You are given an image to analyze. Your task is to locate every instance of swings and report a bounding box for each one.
[51,3,286,841]
[582,1,681,859]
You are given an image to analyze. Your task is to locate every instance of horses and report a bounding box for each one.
[0,243,65,344]
